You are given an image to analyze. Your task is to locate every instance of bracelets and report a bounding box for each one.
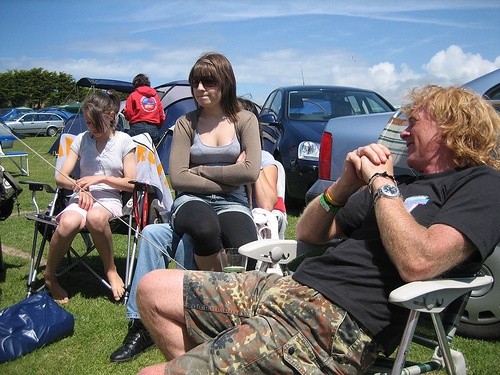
[319,192,341,213]
[325,190,345,207]
[368,171,397,196]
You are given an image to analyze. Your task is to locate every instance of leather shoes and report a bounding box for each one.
[109,319,155,363]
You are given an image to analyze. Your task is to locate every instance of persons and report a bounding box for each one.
[166,53,262,270]
[123,73,166,144]
[135,83,500,375]
[108,96,278,363]
[41,92,137,305]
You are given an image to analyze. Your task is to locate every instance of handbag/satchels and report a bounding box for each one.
[0,289,74,363]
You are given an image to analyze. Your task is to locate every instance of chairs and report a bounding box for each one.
[0,145,30,176]
[238,109,493,375]
[332,100,351,117]
[290,95,304,117]
[19,183,148,306]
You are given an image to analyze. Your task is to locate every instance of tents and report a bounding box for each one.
[48,113,89,156]
[114,80,198,176]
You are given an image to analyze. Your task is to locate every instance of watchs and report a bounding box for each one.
[372,184,401,206]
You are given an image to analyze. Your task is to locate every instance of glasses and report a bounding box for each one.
[189,74,219,88]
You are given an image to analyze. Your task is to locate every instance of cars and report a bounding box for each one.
[259,84,395,206]
[0,100,130,149]
[304,70,499,337]
